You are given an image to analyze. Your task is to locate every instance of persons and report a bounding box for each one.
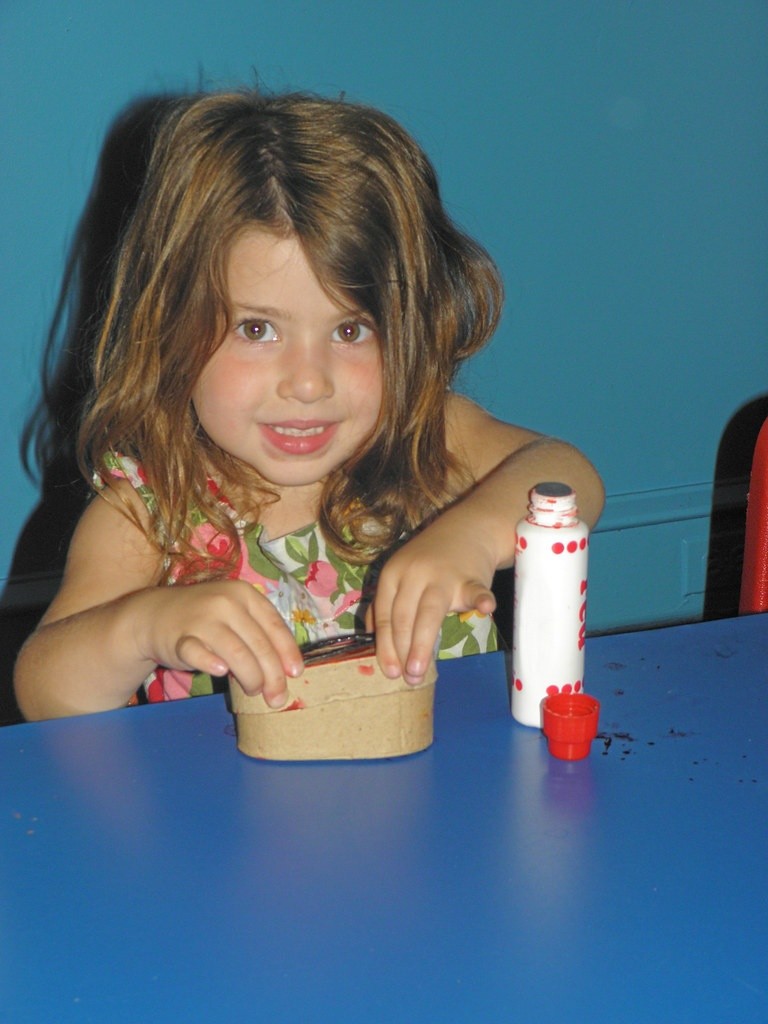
[14,91,605,722]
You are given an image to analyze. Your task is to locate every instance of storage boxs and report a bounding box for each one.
[229,635,441,763]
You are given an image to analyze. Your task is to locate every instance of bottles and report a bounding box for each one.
[511,482,588,728]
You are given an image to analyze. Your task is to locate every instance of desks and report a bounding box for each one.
[0,611,768,1024]
